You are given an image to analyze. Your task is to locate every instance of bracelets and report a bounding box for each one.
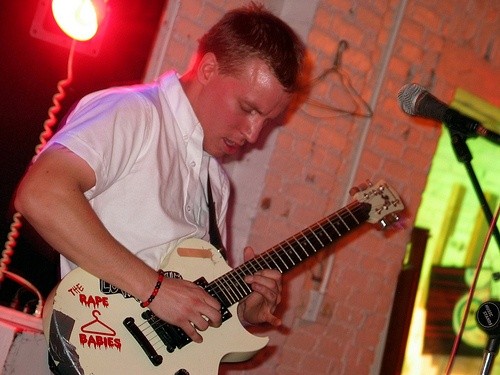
[140,269,164,308]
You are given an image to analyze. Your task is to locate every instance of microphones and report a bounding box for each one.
[396,83,482,138]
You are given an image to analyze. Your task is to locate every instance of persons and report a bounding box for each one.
[11,1,307,344]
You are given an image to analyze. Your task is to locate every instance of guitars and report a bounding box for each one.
[40,179,407,375]
